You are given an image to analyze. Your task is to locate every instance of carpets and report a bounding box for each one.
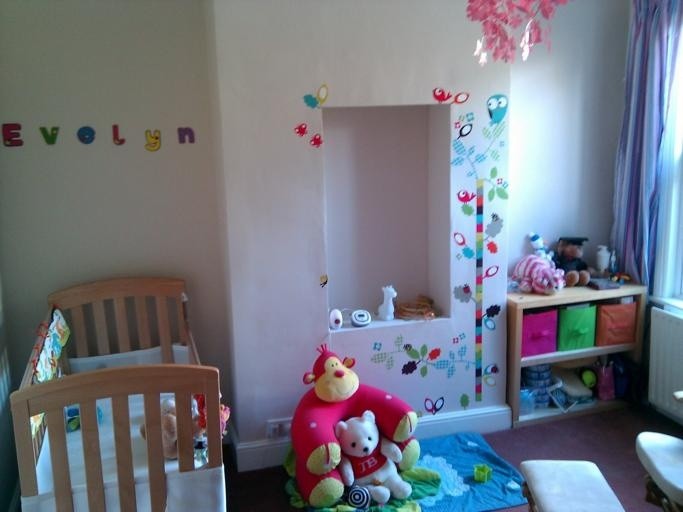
[355,429,528,511]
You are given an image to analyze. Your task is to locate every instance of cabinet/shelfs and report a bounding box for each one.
[506,281,648,429]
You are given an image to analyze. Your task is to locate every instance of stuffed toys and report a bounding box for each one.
[289,342,421,509]
[550,235,592,287]
[332,408,413,504]
[511,254,566,295]
[203,403,230,440]
[138,398,205,461]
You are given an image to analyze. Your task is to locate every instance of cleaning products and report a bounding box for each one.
[610,249,618,274]
[595,245,611,281]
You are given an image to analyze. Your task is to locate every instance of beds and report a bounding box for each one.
[8,277,227,512]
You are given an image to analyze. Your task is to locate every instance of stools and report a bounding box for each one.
[518,458,626,511]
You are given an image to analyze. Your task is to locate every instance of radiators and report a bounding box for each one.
[648,306,683,426]
